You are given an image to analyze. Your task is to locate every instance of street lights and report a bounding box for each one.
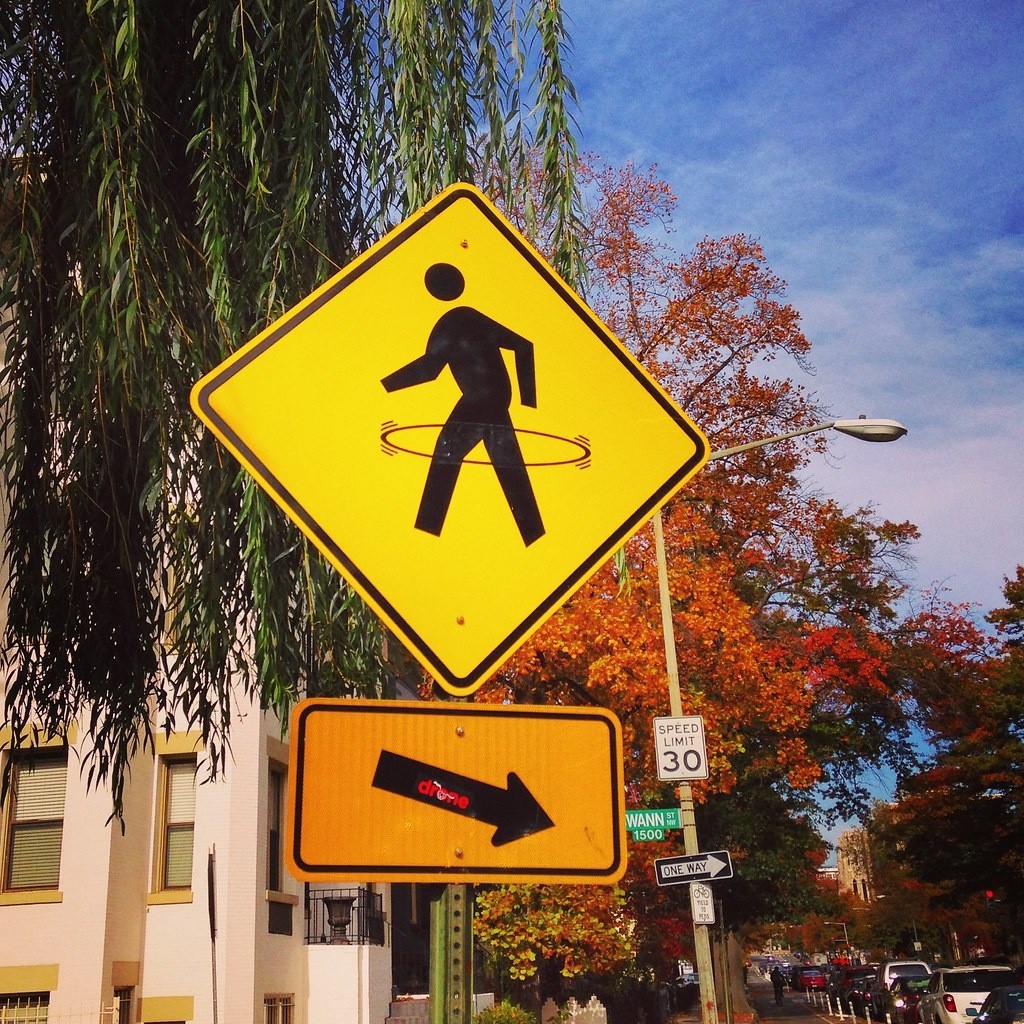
[825,921,849,946]
[652,420,909,1023]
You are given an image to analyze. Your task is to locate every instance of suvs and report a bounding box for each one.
[914,964,1022,1024]
[834,965,877,1008]
[788,965,821,990]
[869,961,933,1014]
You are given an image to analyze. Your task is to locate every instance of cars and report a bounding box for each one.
[846,980,864,1016]
[797,970,827,991]
[859,975,877,1018]
[793,951,812,966]
[880,975,931,1024]
[758,957,792,985]
[967,985,1024,1024]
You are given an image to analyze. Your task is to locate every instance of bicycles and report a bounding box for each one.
[775,981,783,1005]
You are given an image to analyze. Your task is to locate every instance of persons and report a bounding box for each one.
[771,966,787,1005]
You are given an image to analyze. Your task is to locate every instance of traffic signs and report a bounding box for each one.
[626,808,683,842]
[654,850,733,886]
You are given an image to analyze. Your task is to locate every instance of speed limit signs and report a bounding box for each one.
[654,715,709,781]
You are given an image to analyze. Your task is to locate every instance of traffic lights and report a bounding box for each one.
[986,890,994,910]
